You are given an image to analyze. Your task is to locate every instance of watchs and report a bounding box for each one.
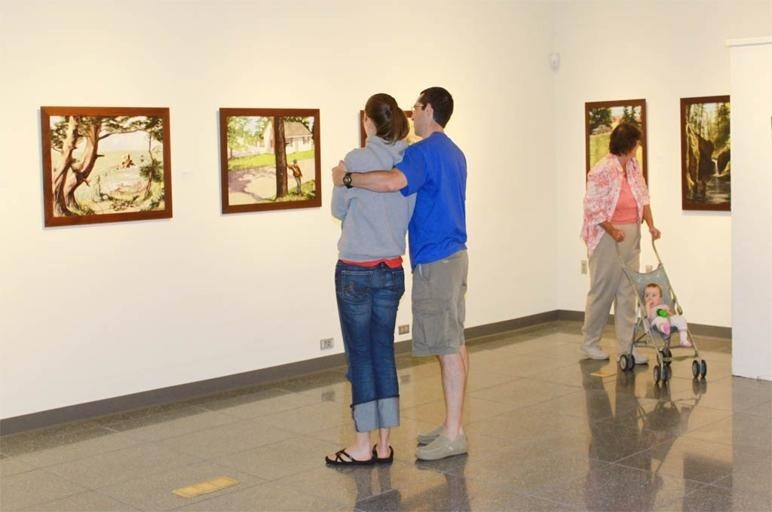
[343,171,351,191]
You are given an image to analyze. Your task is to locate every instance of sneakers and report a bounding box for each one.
[616,349,649,364]
[417,423,468,444]
[578,346,610,360]
[415,435,468,460]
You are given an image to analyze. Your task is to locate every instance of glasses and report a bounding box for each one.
[410,105,424,113]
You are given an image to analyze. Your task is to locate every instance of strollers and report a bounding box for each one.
[615,235,708,383]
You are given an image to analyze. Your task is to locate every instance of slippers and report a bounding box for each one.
[326,448,376,465]
[373,444,393,463]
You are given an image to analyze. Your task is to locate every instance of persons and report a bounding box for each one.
[286,160,302,195]
[643,283,693,347]
[332,88,469,461]
[326,93,418,467]
[578,124,661,365]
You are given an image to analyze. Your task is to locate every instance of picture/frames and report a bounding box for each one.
[680,94,731,211]
[585,98,649,188]
[360,111,422,147]
[40,106,173,227]
[220,108,322,214]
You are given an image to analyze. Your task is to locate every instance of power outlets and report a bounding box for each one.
[320,338,334,349]
